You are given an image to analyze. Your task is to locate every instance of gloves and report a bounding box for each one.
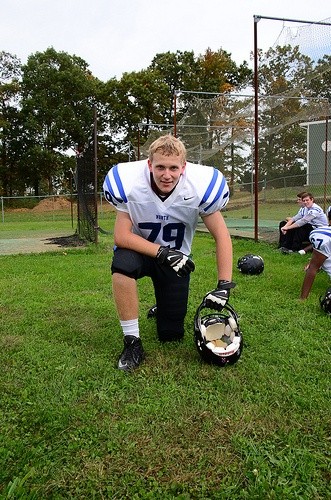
[204,280,237,311]
[155,245,196,278]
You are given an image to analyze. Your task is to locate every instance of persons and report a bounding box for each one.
[278,192,329,254]
[103,135,233,370]
[298,226,331,300]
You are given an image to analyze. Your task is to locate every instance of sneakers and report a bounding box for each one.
[117,335,145,370]
[147,306,158,318]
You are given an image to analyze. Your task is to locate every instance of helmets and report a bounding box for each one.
[238,254,264,275]
[195,315,242,366]
